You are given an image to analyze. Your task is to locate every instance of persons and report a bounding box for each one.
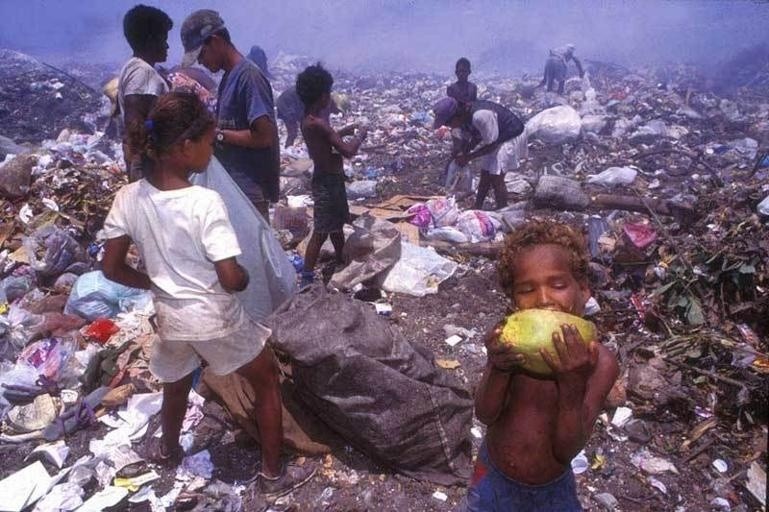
[100,92,319,496]
[432,58,528,212]
[118,6,173,185]
[181,10,280,226]
[464,221,620,512]
[246,45,269,76]
[276,62,367,291]
[542,44,584,95]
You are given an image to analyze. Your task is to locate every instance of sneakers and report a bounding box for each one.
[300,277,315,288]
[258,462,318,497]
[144,438,183,467]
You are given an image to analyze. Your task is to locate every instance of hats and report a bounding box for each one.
[180,9,226,70]
[432,96,458,129]
